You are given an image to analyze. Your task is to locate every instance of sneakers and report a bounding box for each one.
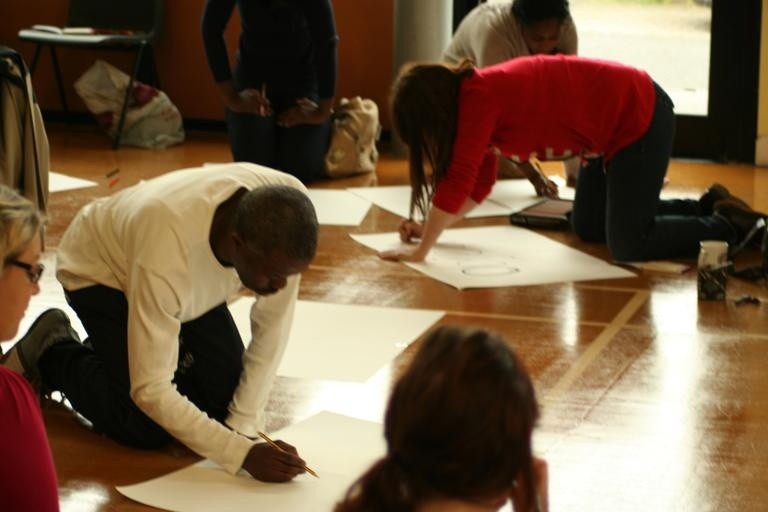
[1,310,77,385]
[698,182,768,240]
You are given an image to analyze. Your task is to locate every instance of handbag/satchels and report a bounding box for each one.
[325,96,380,176]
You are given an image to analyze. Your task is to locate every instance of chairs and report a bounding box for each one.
[18,0,165,151]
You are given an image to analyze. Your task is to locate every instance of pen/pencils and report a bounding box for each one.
[535,161,560,199]
[257,431,319,478]
[407,191,415,243]
[106,168,121,187]
[260,82,267,118]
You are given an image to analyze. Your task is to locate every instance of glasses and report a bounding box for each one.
[10,258,45,283]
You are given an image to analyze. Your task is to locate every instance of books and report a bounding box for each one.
[61,27,135,36]
[32,24,62,34]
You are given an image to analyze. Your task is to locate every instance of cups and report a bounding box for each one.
[695,241,731,302]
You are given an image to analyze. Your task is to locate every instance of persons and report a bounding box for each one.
[330,322,552,511]
[0,184,62,512]
[439,1,581,202]
[0,161,318,484]
[375,53,768,264]
[202,1,339,187]
